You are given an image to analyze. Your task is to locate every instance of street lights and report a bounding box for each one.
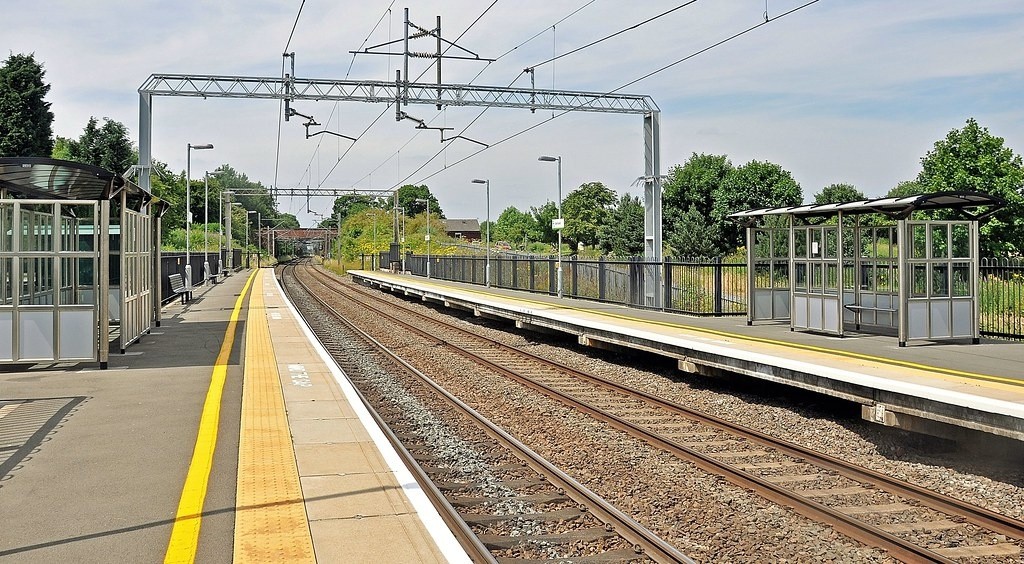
[472,179,491,288]
[218,191,235,274]
[538,156,563,298]
[204,171,225,281]
[416,198,431,280]
[246,211,257,268]
[393,207,405,275]
[366,213,376,270]
[185,143,214,290]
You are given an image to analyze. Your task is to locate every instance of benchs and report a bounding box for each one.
[168,273,197,305]
[222,270,229,277]
[210,275,219,284]
[844,304,898,313]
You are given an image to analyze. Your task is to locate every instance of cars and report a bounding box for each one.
[7,272,36,290]
[496,241,512,251]
